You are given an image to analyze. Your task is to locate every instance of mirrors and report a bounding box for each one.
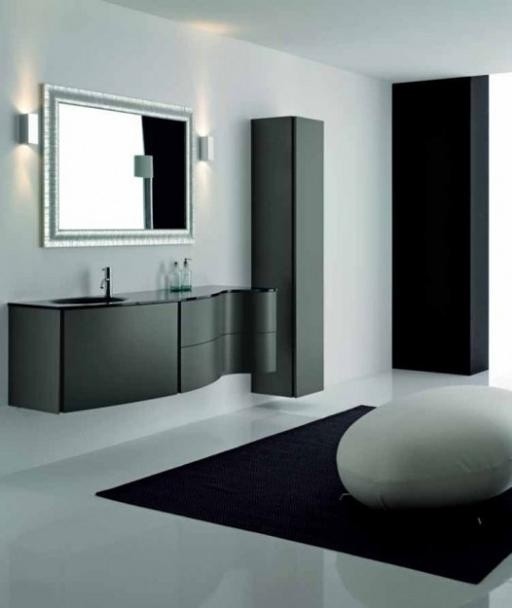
[42,83,195,247]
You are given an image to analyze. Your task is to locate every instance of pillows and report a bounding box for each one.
[336,386,512,506]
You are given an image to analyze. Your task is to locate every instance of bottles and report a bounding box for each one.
[170,258,191,292]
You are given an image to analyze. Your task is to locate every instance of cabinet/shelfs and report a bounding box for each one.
[6,288,277,414]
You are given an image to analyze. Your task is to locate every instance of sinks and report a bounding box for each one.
[53,295,125,305]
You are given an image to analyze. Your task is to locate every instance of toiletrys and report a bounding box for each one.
[168,261,181,291]
[181,257,193,291]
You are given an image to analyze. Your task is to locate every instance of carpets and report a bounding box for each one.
[95,405,512,585]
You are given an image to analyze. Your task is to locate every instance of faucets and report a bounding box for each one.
[99,265,111,296]
